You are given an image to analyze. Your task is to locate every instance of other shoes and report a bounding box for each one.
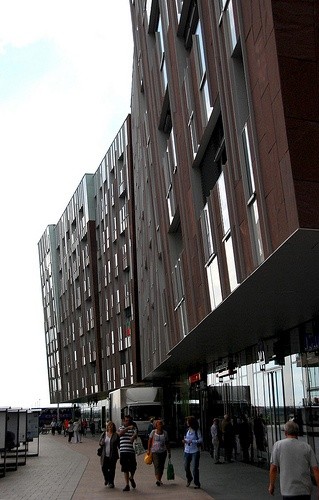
[109,484,114,488]
[130,477,136,488]
[193,484,200,489]
[156,480,161,486]
[122,484,130,491]
[105,480,108,485]
[186,478,193,487]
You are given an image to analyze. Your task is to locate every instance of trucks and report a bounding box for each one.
[109,387,162,449]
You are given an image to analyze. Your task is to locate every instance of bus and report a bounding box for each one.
[31,406,81,425]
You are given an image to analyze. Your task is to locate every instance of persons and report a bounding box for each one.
[99,422,120,488]
[89,420,96,437]
[210,418,225,464]
[81,419,88,437]
[181,416,204,489]
[235,412,269,463]
[51,419,57,435]
[118,418,140,491]
[73,418,84,444]
[268,422,319,500]
[62,419,74,443]
[57,418,63,435]
[97,420,103,434]
[148,421,173,487]
[222,415,236,462]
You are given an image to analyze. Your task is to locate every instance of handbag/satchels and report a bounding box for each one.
[132,437,146,456]
[145,452,153,465]
[167,460,175,480]
[97,447,102,456]
